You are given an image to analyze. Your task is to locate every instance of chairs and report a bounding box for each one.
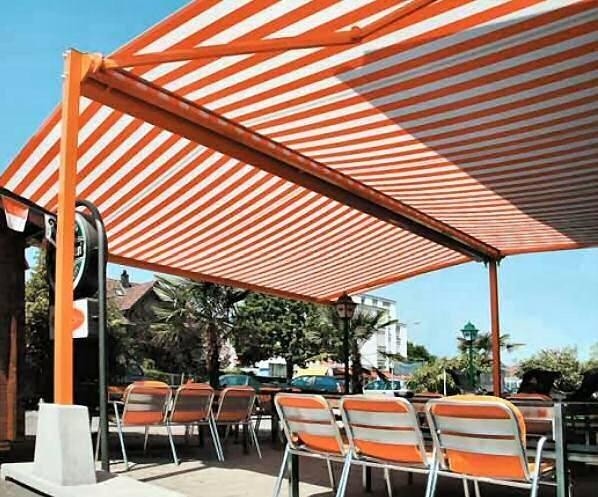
[163,381,225,463]
[412,392,441,402]
[274,392,355,497]
[189,384,262,461]
[428,395,557,497]
[254,386,304,445]
[339,396,434,496]
[95,382,179,473]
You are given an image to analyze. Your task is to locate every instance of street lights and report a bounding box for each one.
[332,291,359,394]
[460,320,480,394]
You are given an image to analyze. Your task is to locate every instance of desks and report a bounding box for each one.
[101,393,356,473]
[288,400,598,497]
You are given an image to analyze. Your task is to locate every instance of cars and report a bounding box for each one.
[203,373,423,413]
